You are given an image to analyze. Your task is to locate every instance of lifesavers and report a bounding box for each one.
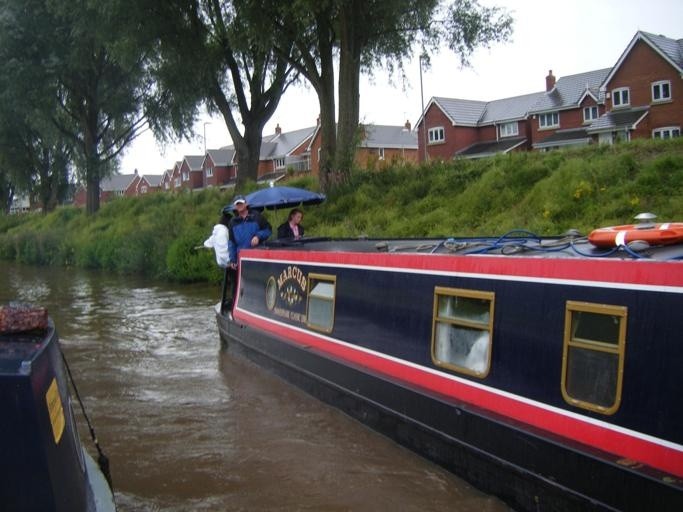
[587,223,683,247]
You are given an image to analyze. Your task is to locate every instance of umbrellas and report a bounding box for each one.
[223,183,327,225]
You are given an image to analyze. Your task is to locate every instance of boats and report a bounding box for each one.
[1,303,119,512]
[213,220,682,510]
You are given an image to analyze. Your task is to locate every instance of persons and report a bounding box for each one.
[277,208,305,239]
[202,213,231,314]
[227,194,272,311]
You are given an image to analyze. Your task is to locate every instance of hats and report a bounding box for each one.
[234,195,245,205]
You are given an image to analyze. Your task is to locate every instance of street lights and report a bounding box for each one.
[203,122,211,157]
[419,53,431,164]
